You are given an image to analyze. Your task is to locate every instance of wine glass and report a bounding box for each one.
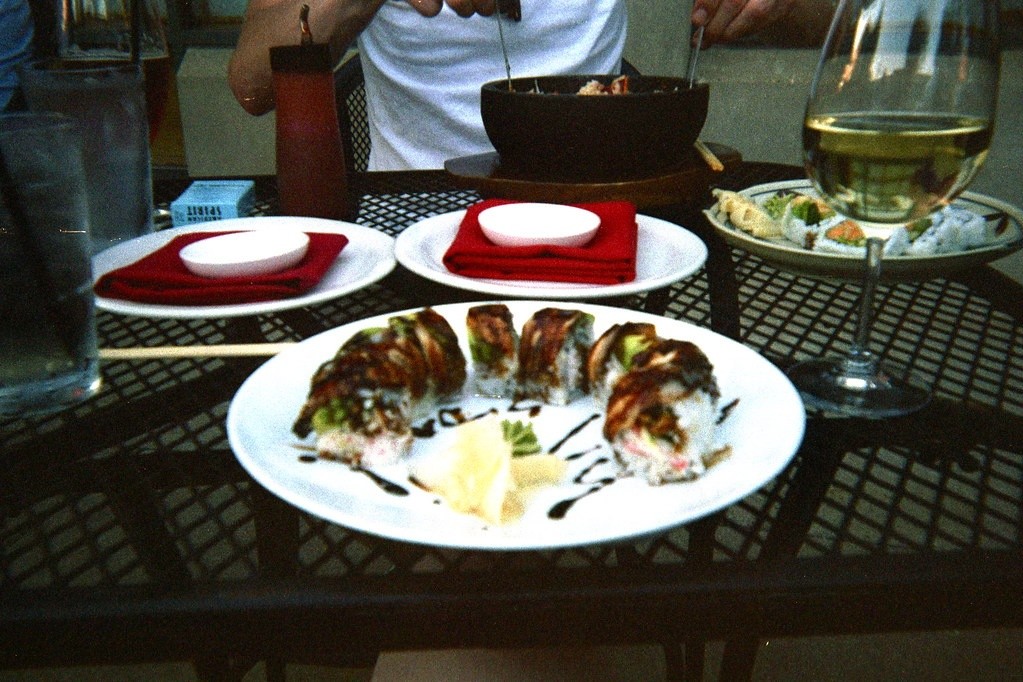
[781,0,1001,419]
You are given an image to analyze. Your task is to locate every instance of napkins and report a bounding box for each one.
[443,200,635,284]
[94,231,348,306]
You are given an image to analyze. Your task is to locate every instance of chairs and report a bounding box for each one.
[266,42,743,680]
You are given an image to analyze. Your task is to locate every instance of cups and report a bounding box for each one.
[269,41,351,221]
[0,113,102,422]
[23,56,156,256]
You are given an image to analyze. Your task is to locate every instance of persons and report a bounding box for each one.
[229,0,850,171]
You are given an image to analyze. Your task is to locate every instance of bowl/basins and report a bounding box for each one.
[443,141,742,214]
[179,229,310,276]
[480,74,709,183]
[477,202,602,247]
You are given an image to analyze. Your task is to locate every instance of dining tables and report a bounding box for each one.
[0,174,1022,682]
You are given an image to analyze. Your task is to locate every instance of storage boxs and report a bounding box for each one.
[171,179,256,228]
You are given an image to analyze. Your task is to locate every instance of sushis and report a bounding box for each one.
[283,305,726,486]
[778,195,986,257]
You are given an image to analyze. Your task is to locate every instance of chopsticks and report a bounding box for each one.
[694,138,725,171]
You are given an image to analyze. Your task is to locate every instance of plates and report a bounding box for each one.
[707,179,1023,278]
[394,210,709,300]
[225,300,807,553]
[91,215,397,319]
